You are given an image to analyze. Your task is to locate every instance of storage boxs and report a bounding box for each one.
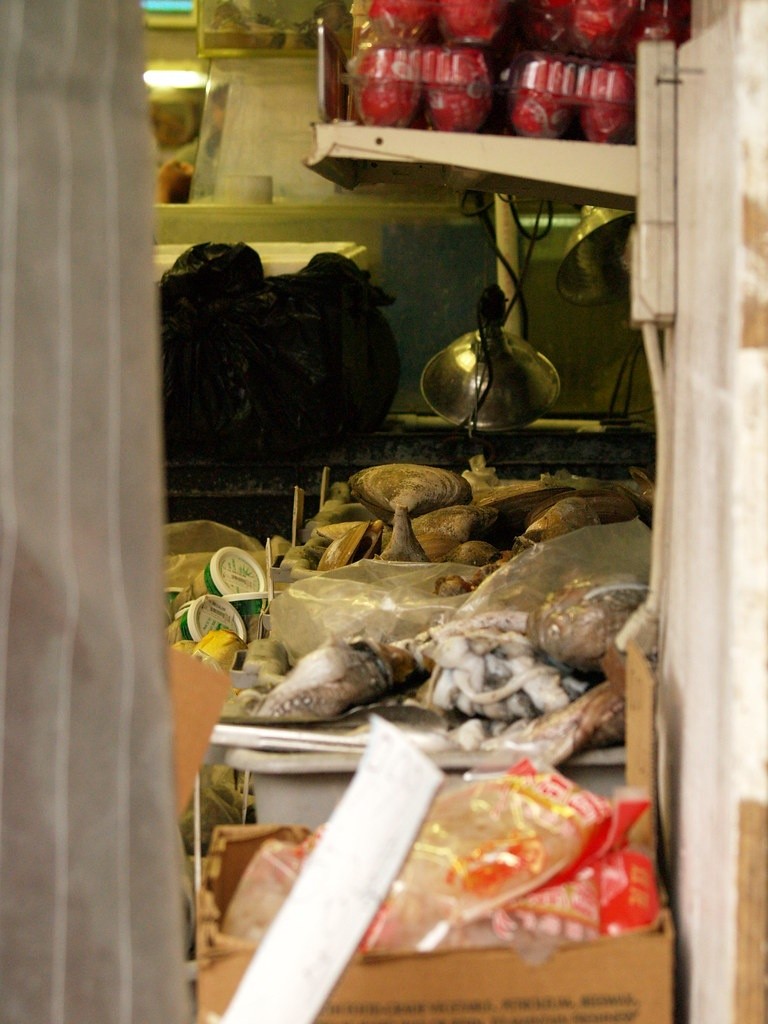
[226,745,627,823]
[195,792,676,1023]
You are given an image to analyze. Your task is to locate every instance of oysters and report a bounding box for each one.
[322,463,655,596]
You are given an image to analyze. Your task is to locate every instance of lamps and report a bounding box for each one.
[419,282,562,433]
[557,204,635,306]
